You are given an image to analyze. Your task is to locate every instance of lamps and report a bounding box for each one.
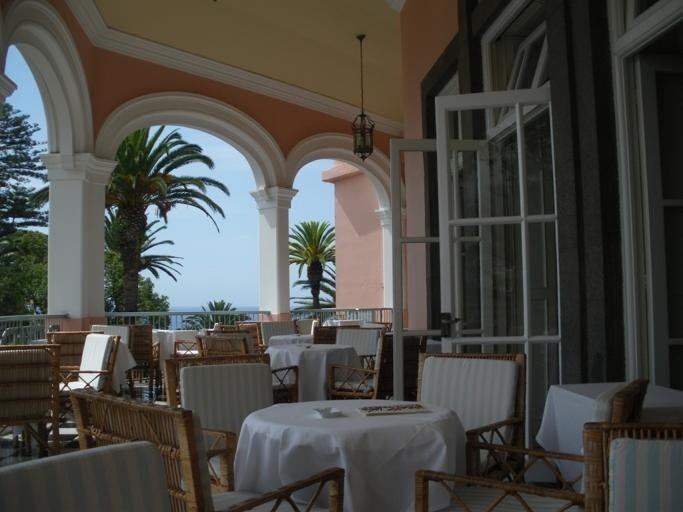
[351,35,375,163]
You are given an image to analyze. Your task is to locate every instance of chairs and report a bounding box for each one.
[0,321,683,512]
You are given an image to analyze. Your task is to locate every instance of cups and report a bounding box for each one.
[297,336,303,346]
[303,335,313,348]
